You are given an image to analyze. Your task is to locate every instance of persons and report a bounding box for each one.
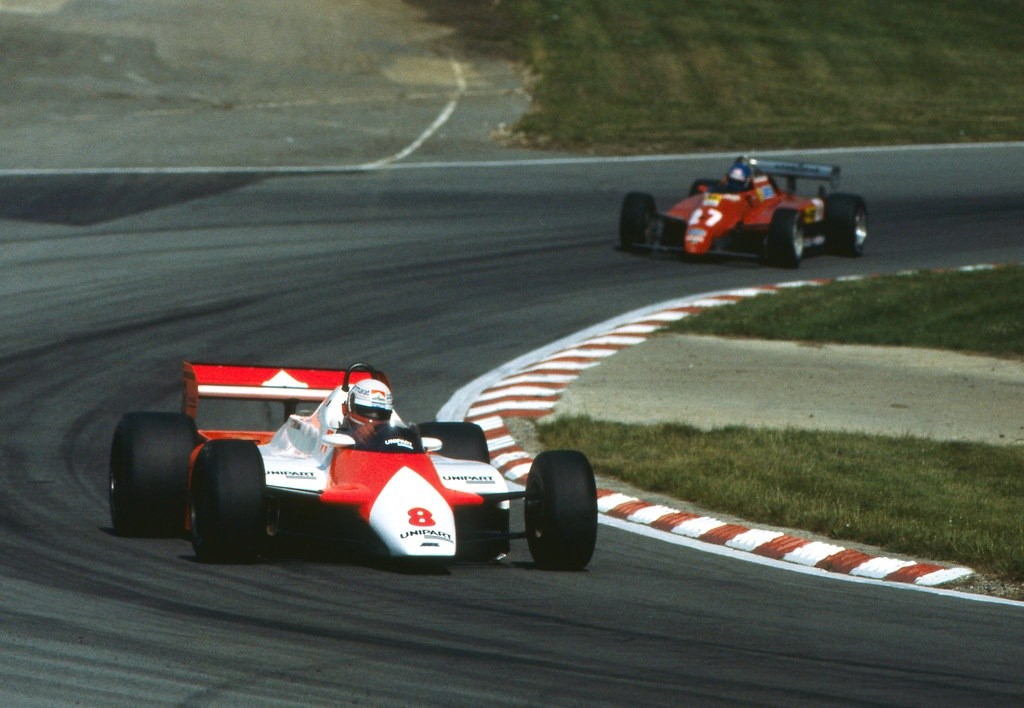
[347,378,394,446]
[726,164,751,188]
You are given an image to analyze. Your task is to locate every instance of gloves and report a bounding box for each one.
[353,424,376,444]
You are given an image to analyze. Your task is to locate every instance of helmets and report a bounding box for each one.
[727,165,752,188]
[347,379,393,428]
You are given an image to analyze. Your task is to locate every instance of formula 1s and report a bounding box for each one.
[101,355,605,577]
[614,156,879,270]
